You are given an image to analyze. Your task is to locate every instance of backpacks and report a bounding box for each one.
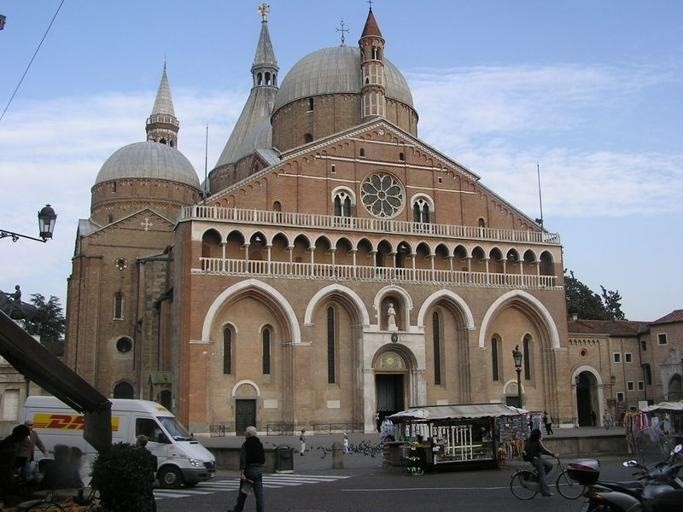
[521,440,534,462]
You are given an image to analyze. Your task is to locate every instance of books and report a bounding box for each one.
[240,478,254,494]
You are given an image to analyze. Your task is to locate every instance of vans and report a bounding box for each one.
[20,396,217,488]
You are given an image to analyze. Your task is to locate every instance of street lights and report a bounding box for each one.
[0,204,58,243]
[513,345,522,408]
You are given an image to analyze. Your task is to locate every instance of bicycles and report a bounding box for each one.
[510,457,585,500]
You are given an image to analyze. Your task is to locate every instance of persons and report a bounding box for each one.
[383,418,395,442]
[524,428,557,498]
[542,411,556,437]
[386,302,397,325]
[0,419,86,505]
[297,428,306,457]
[475,426,490,442]
[526,412,544,431]
[132,434,158,474]
[603,408,612,431]
[378,415,387,443]
[226,425,267,512]
[590,410,597,426]
[343,431,349,455]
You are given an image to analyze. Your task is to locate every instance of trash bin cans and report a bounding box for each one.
[275,445,295,473]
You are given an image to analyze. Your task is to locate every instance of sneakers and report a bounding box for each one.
[542,492,555,497]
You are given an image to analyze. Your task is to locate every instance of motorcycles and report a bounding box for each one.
[567,444,683,512]
